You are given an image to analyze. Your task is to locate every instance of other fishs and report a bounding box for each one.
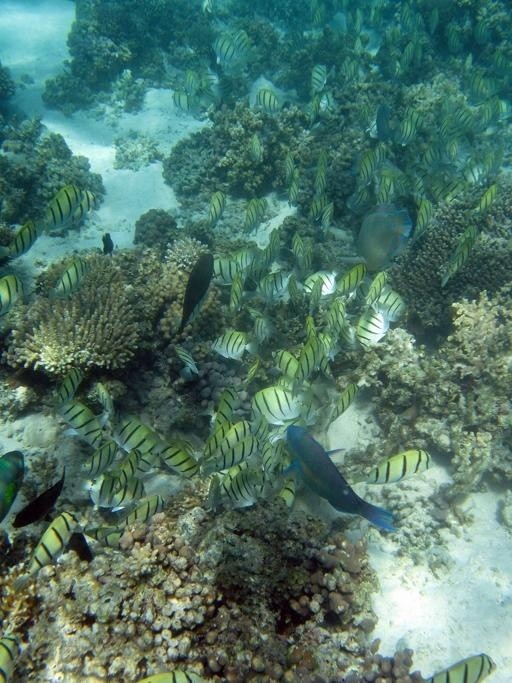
[1,0,511,531]
